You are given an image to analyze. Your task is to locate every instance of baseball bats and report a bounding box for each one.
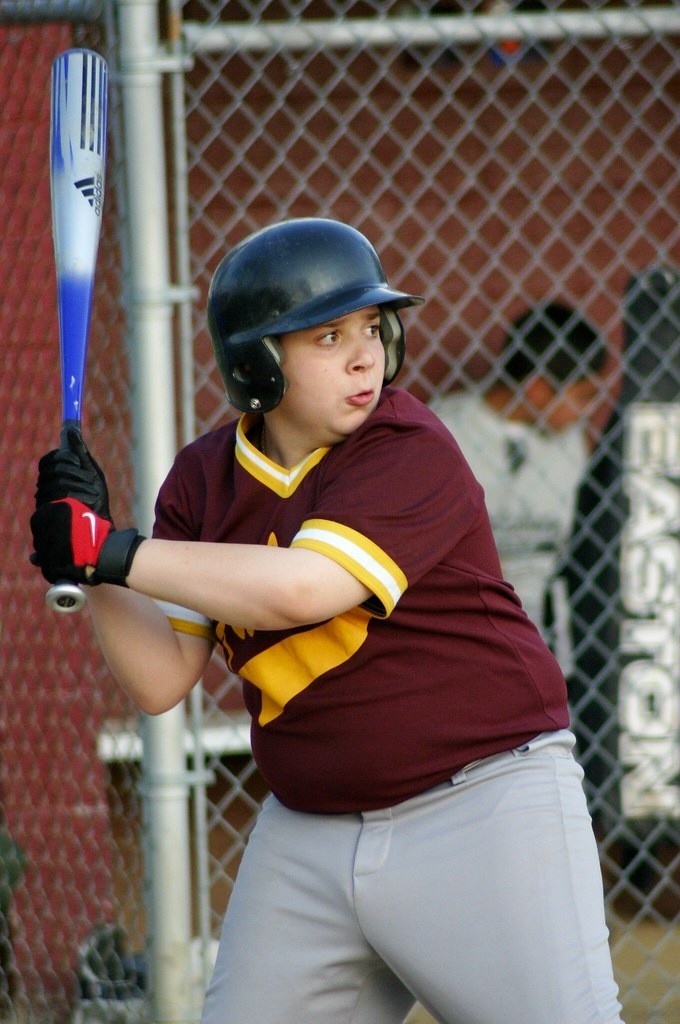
[46,46,109,614]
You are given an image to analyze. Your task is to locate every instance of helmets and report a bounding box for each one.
[207,218,426,418]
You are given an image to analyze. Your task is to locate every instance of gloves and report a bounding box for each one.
[34,426,115,531]
[29,499,146,590]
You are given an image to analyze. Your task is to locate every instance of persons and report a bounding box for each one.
[422,300,612,644]
[31,219,623,1024]
